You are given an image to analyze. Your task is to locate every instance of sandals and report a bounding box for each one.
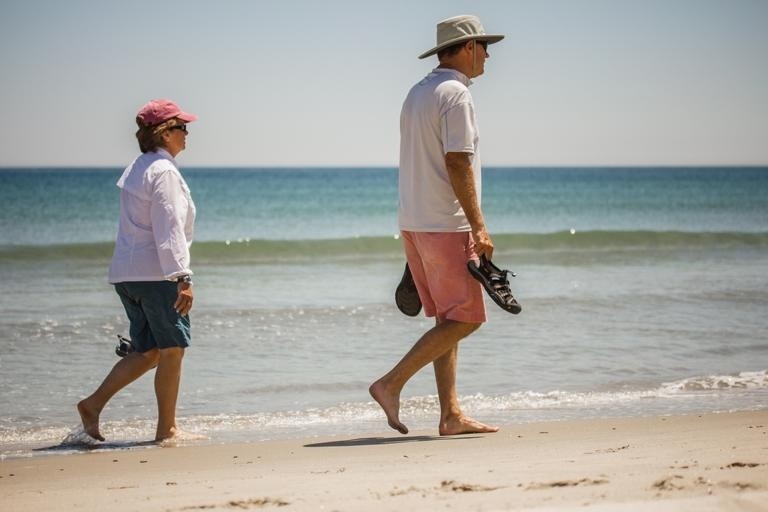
[468,254,521,315]
[394,262,421,317]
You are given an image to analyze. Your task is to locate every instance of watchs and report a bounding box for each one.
[177,275,193,285]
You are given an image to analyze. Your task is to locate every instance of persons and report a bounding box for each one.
[367,12,506,438]
[75,98,197,444]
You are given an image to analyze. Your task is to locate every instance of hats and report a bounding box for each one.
[136,99,197,126]
[417,14,505,60]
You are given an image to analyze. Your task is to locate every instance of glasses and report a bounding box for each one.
[164,124,186,132]
